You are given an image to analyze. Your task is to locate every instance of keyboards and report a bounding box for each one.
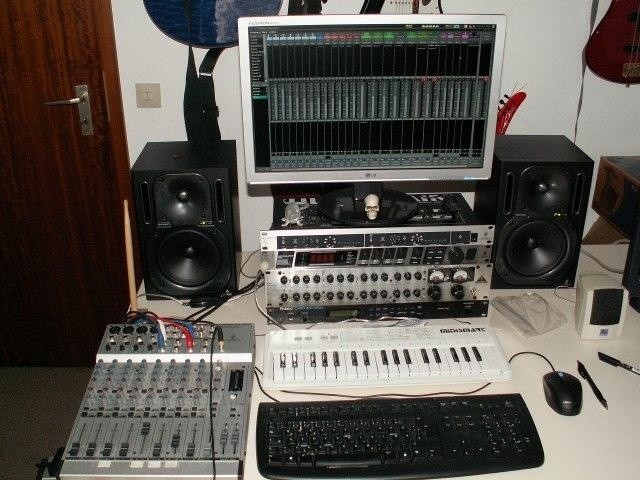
[257,393,544,480]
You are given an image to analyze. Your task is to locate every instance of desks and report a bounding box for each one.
[124,241,639,478]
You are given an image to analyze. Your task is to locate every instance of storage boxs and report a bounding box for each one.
[589,154,640,243]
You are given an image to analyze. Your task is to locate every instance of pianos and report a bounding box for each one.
[261,329,512,388]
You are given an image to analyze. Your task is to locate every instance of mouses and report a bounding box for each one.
[542,371,582,415]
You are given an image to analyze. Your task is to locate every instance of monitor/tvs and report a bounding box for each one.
[239,14,506,225]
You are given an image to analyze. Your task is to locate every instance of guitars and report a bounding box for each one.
[582,1,639,84]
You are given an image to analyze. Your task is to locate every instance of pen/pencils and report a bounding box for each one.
[577,360,608,410]
[598,352,640,376]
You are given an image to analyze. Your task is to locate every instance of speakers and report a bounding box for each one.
[130,140,241,301]
[473,136,594,289]
